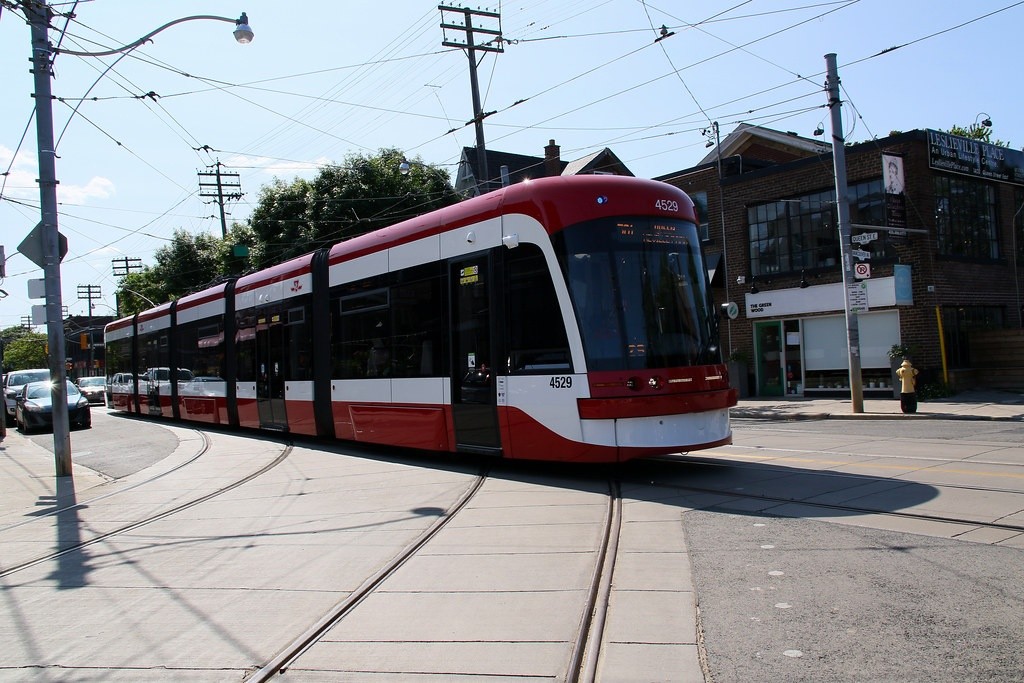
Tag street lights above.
[30,12,254,477]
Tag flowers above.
[886,343,911,360]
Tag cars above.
[109,367,195,395]
[183,377,226,397]
[7,380,91,433]
[3,369,50,416]
[75,377,105,404]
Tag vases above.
[890,358,904,399]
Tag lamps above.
[972,113,992,142]
[749,277,772,295]
[800,269,820,290]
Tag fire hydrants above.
[895,359,919,412]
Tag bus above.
[104,176,738,464]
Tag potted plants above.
[887,379,892,388]
[819,378,885,388]
